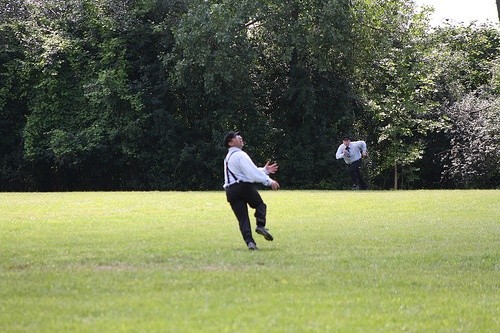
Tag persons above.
[336,136,368,190]
[223,130,281,248]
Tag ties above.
[345,146,350,157]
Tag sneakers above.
[255,226,273,241]
[247,241,257,250]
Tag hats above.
[224,130,240,145]
